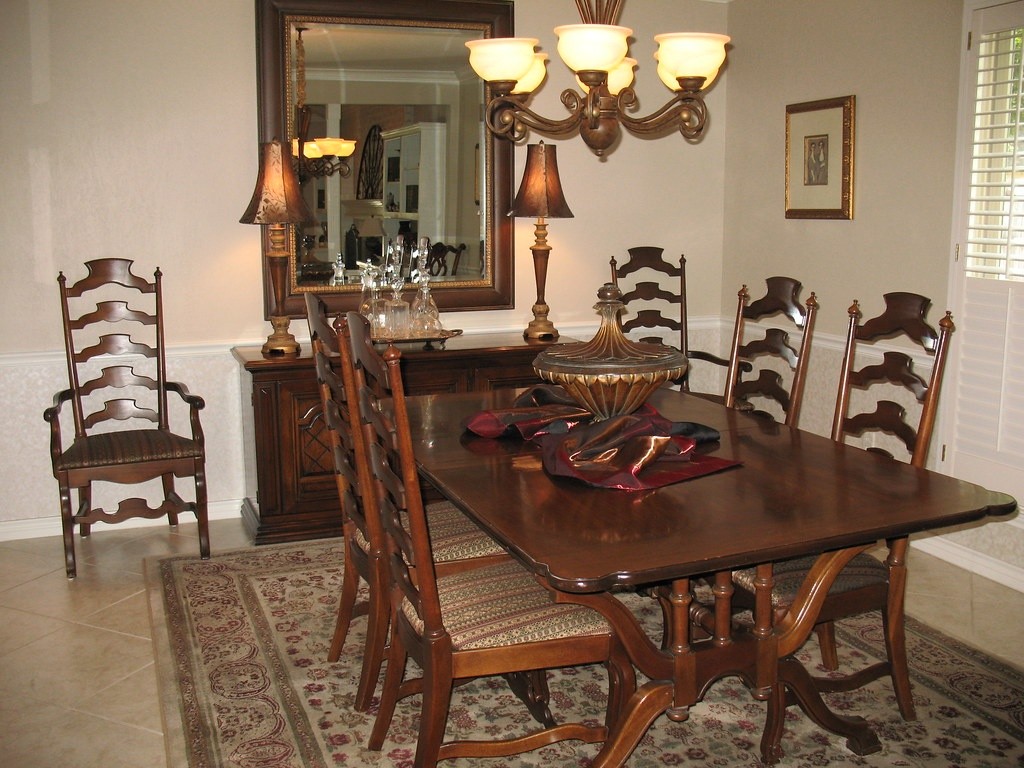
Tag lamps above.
[238,141,317,357]
[465,0,730,156]
[507,139,575,339]
[292,136,357,187]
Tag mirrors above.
[285,14,495,296]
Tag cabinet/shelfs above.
[379,122,447,267]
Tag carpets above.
[143,536,1023,768]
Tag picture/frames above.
[785,95,854,220]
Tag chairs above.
[385,242,466,283]
[689,277,820,640]
[730,292,953,766]
[44,257,210,578]
[305,287,549,713]
[343,311,638,768]
[610,245,755,415]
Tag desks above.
[376,387,1019,768]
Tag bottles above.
[357,273,442,339]
